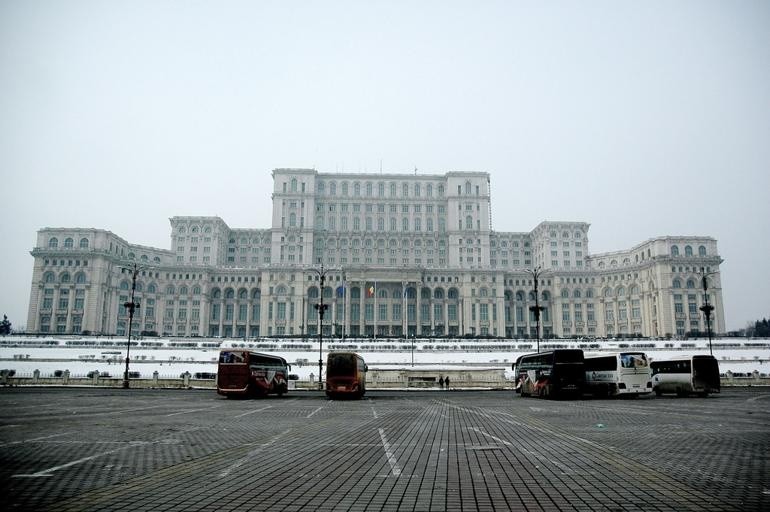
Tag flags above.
[336,286,344,295]
[368,286,375,297]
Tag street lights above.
[523,264,553,354]
[410,332,415,368]
[696,265,723,355]
[115,263,156,388]
[304,263,341,389]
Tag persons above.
[439,377,444,388]
[445,377,449,388]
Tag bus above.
[720,370,752,378]
[650,355,720,400]
[511,349,585,399]
[215,350,291,400]
[327,352,367,399]
[407,376,437,389]
[583,352,653,398]
